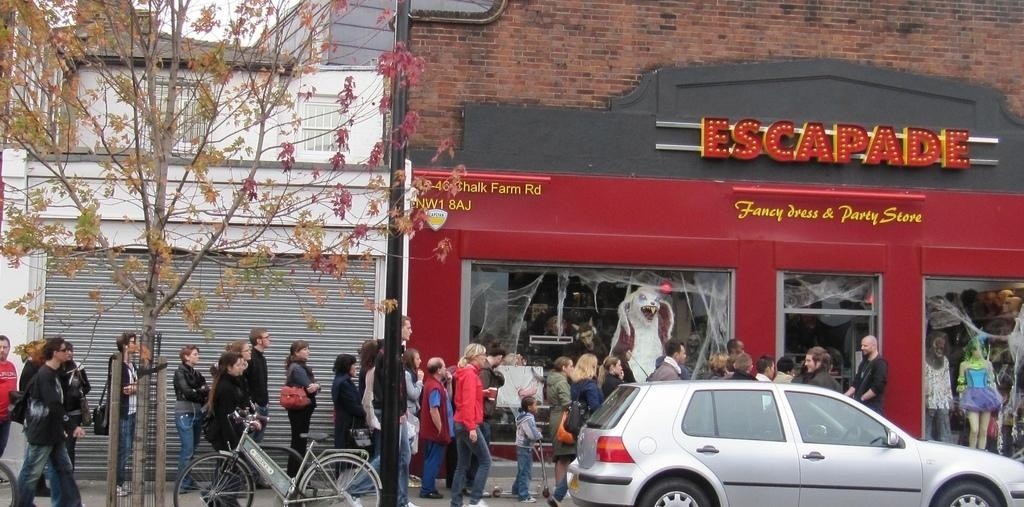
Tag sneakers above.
[116,483,131,497]
[419,491,443,499]
[342,491,363,507]
[401,502,420,507]
[450,489,491,507]
[520,496,535,505]
[547,497,561,507]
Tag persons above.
[647,338,841,393]
[546,342,635,505]
[287,340,321,487]
[844,335,888,414]
[956,337,1003,450]
[107,331,138,495]
[512,397,543,502]
[923,330,955,444]
[331,317,505,506]
[174,345,210,491]
[1,334,90,506]
[199,328,270,507]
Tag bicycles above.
[0,459,23,507]
[171,393,386,507]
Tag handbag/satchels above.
[349,413,371,448]
[280,384,311,409]
[556,400,591,445]
[200,401,211,439]
[7,389,30,425]
[987,413,999,439]
[92,403,111,436]
[80,396,92,427]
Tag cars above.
[475,356,554,444]
[563,377,1024,507]
